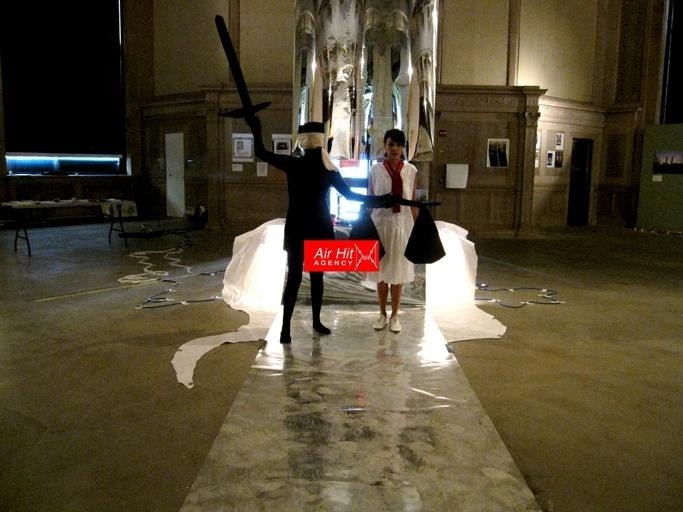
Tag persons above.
[244,114,398,344]
[282,331,331,507]
[365,129,419,333]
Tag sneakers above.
[389,315,402,332]
[373,314,389,330]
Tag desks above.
[1,196,138,258]
